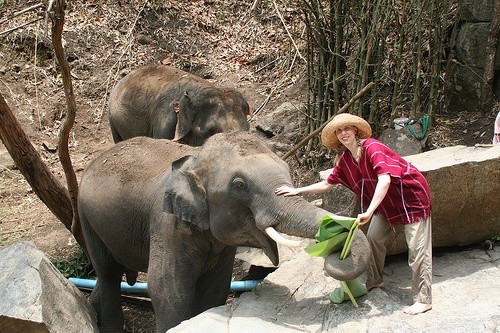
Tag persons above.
[274,113,434,316]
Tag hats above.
[320,113,372,150]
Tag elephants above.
[77,129,372,333]
[108,63,251,147]
